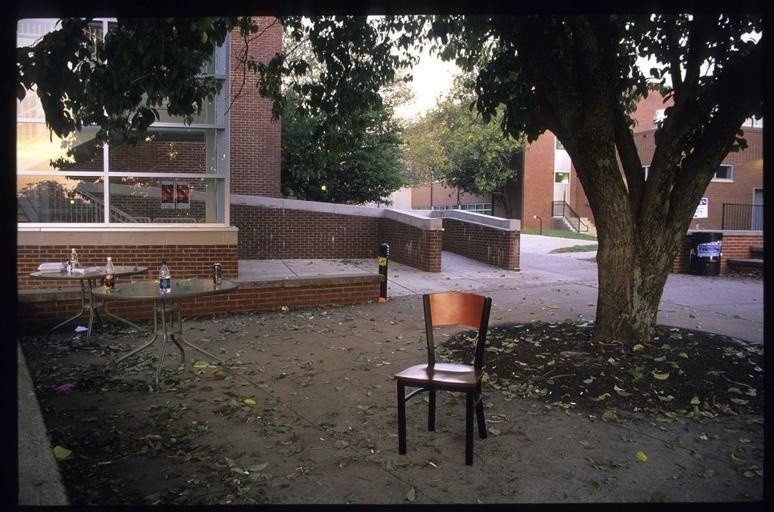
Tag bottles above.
[105,256,116,291]
[158,260,172,295]
[213,262,222,286]
[70,247,80,275]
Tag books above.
[37,262,66,273]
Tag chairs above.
[394,289,498,467]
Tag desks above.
[29,262,239,393]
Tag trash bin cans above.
[691,232,722,276]
